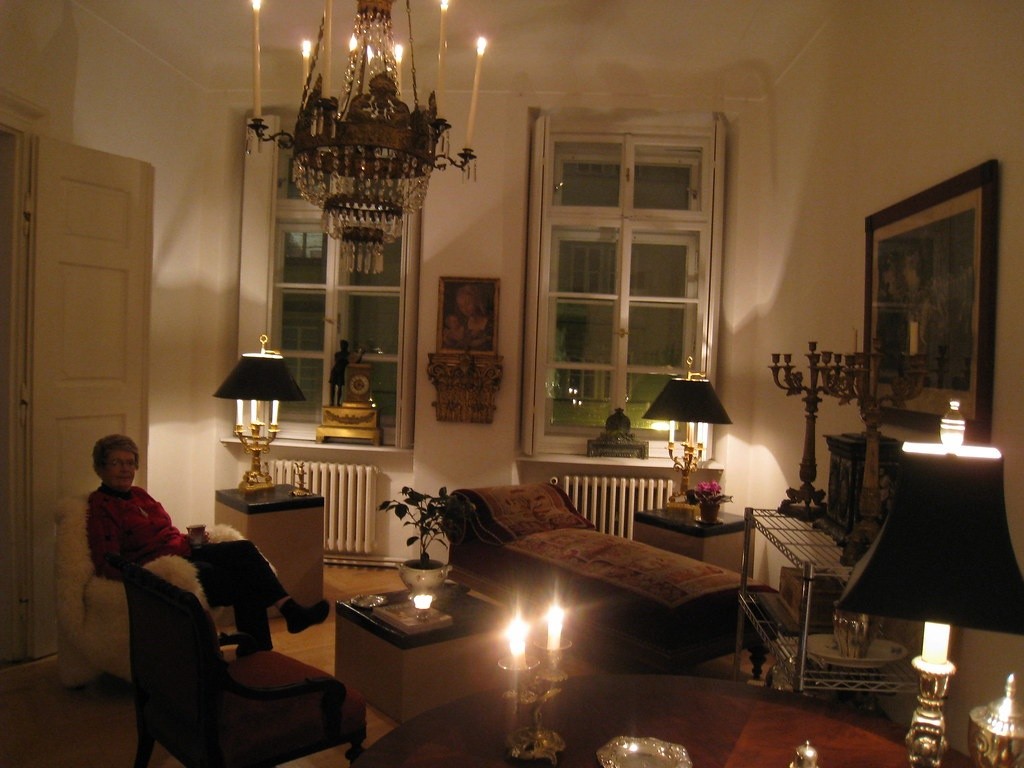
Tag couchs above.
[438,481,777,668]
[56,503,281,694]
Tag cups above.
[186,525,207,548]
[832,612,883,658]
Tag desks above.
[352,668,926,767]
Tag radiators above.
[258,458,380,557]
[547,476,676,542]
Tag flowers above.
[700,478,723,498]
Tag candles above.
[909,319,918,355]
[408,592,436,622]
[851,327,857,354]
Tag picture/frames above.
[861,157,1002,445]
[436,274,503,360]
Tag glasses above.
[106,458,136,468]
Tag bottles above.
[940,401,966,449]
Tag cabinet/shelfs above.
[730,504,943,702]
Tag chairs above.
[117,559,368,768]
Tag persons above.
[443,284,493,349]
[329,340,349,407]
[86,434,330,659]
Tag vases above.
[697,501,726,528]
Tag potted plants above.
[377,487,472,605]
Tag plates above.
[796,634,909,668]
[596,736,692,768]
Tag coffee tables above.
[334,589,497,726]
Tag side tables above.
[211,483,325,621]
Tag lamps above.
[242,2,487,275]
[494,584,574,767]
[212,331,307,495]
[835,398,1023,767]
[642,354,733,522]
